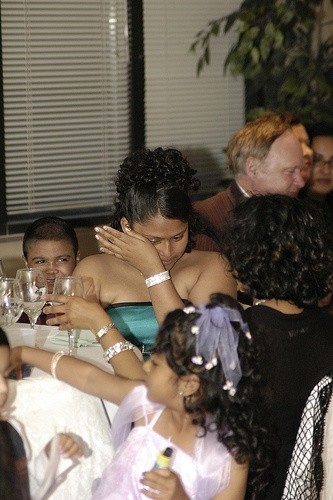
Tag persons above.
[188,113,333,303]
[5,293,281,500]
[43,193,333,500]
[281,353,333,500]
[16,216,81,325]
[60,146,238,362]
[0,326,80,500]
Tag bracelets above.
[145,269,172,292]
[102,340,134,364]
[49,352,63,379]
[94,321,116,341]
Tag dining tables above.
[0,323,145,500]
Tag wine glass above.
[14,268,48,348]
[0,278,24,329]
[52,276,83,357]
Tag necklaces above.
[254,297,267,305]
